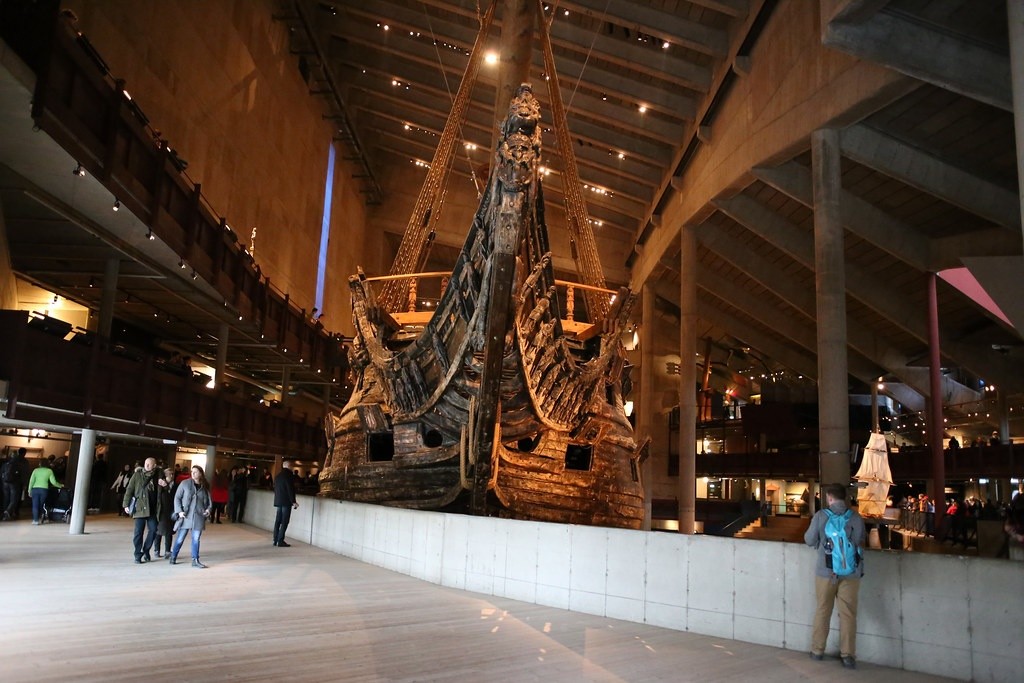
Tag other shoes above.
[170,558,176,565]
[842,656,856,669]
[2,511,11,521]
[143,547,151,562]
[273,541,291,547]
[164,551,172,559]
[32,520,39,525]
[216,520,222,524]
[210,517,215,523]
[809,651,823,660]
[155,551,160,558]
[134,558,142,564]
[191,559,205,568]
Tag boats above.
[318,82,646,533]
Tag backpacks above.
[821,507,864,575]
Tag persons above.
[262,466,321,491]
[804,481,866,669]
[886,431,1024,516]
[0,446,252,565]
[273,461,296,548]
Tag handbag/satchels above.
[171,512,179,521]
